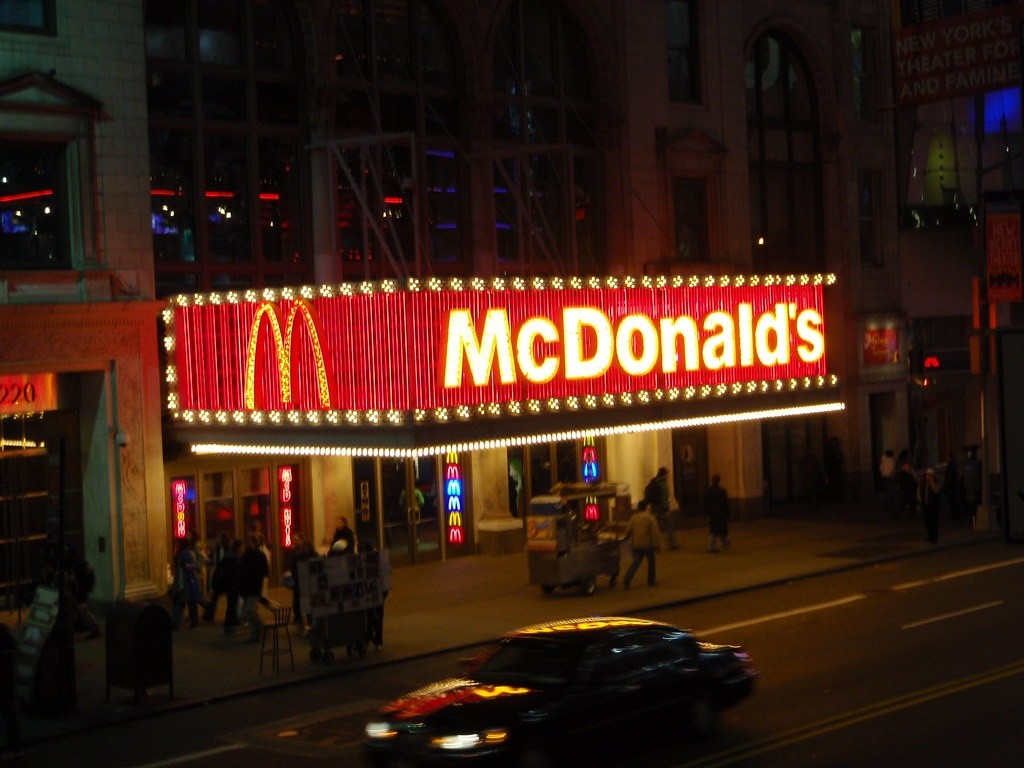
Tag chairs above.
[259,608,295,676]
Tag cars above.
[364,615,758,768]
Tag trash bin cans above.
[105,601,174,704]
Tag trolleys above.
[295,552,392,660]
[523,482,634,594]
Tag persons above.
[509,467,518,518]
[623,498,661,591]
[645,467,681,551]
[44,544,102,641]
[924,462,941,543]
[399,478,424,545]
[704,474,732,554]
[0,623,23,759]
[166,516,385,652]
[798,436,1004,517]
